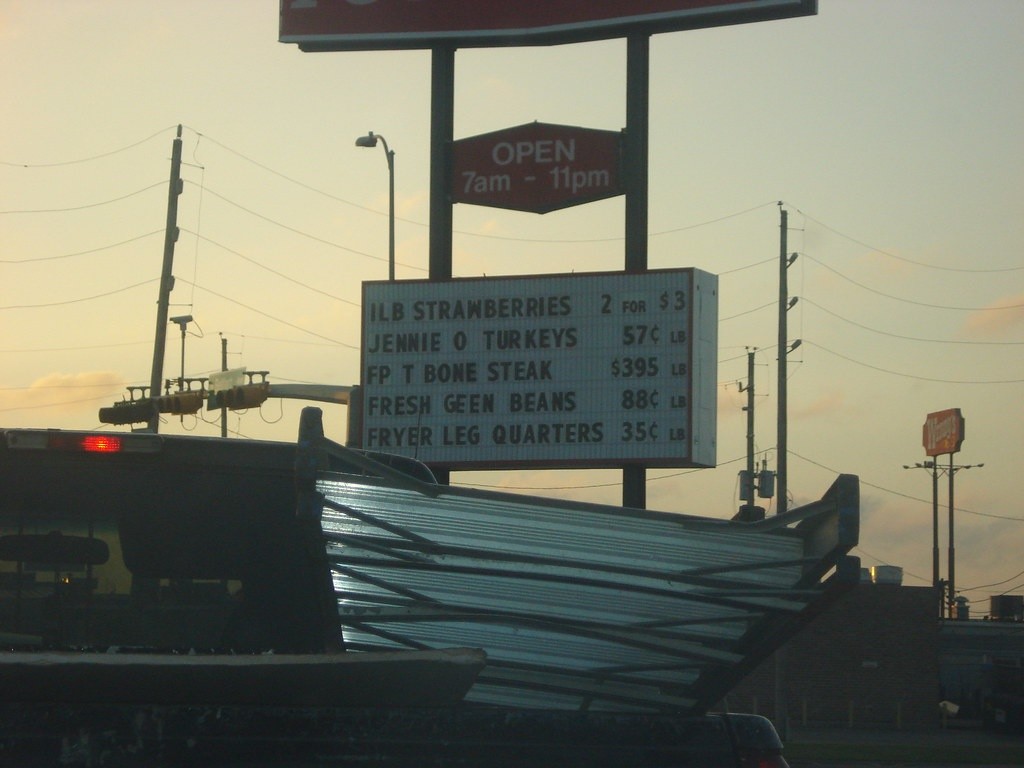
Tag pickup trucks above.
[1,404,795,767]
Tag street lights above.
[354,130,397,279]
